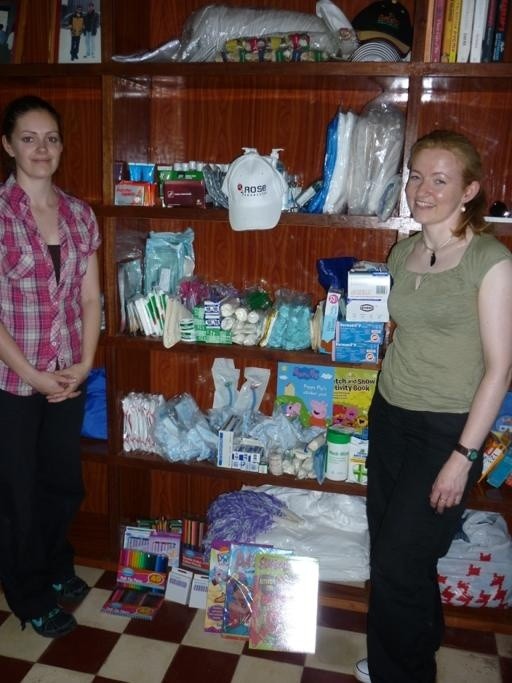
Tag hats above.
[351,0,413,59]
[221,153,289,231]
[316,1,360,61]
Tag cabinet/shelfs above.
[0,0,111,575]
[404,0,512,634]
[100,0,420,609]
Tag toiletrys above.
[127,160,229,209]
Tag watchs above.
[454,442,479,462]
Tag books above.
[423,0,508,63]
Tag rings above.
[438,498,447,504]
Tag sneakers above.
[25,601,77,638]
[353,658,372,683]
[49,576,90,608]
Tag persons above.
[1,97,103,637]
[351,128,511,683]
[67,4,87,61]
[83,3,100,58]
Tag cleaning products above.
[266,145,292,211]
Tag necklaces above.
[422,235,452,266]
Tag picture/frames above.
[54,0,101,64]
[0,0,28,64]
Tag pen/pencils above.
[122,537,176,573]
[182,555,209,570]
[194,585,208,592]
[152,514,168,534]
[104,575,164,608]
